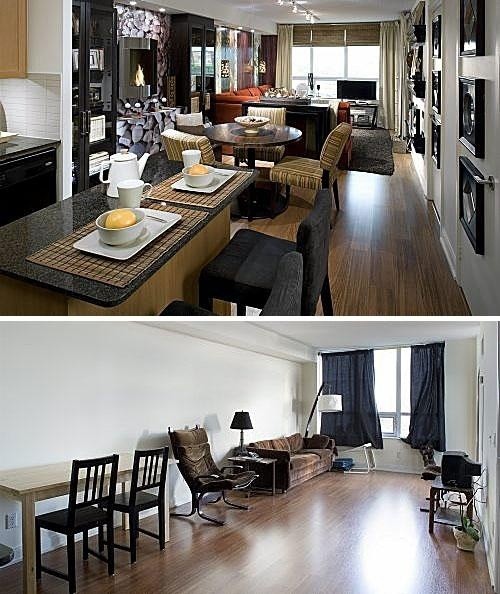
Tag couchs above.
[232,433,337,492]
[215,84,353,168]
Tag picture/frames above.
[431,0,486,255]
[73,48,100,69]
[89,115,105,142]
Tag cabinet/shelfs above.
[171,13,216,122]
[0,148,58,227]
[71,0,117,192]
[1,0,27,77]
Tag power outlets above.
[7,512,17,528]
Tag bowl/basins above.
[181,166,218,189]
[94,208,146,248]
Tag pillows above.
[307,434,329,449]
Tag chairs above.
[160,127,215,162]
[269,121,352,228]
[34,452,120,594]
[168,424,260,524]
[176,111,223,150]
[97,444,168,564]
[159,251,305,314]
[233,105,286,191]
[199,187,333,315]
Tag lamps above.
[277,0,315,25]
[259,34,266,72]
[221,28,230,76]
[305,382,342,436]
[229,410,253,453]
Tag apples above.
[106,209,135,229]
[187,164,208,175]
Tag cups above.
[181,149,201,166]
[117,179,154,211]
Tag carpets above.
[337,126,394,174]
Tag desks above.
[0,453,179,591]
[428,476,473,532]
[206,122,303,219]
[227,456,277,498]
[350,103,378,129]
[0,158,260,315]
[241,99,332,164]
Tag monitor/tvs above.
[337,80,376,100]
[441,451,481,487]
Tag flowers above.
[440,488,481,542]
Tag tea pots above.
[97,149,151,199]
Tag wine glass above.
[317,85,321,97]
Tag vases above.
[452,524,481,552]
